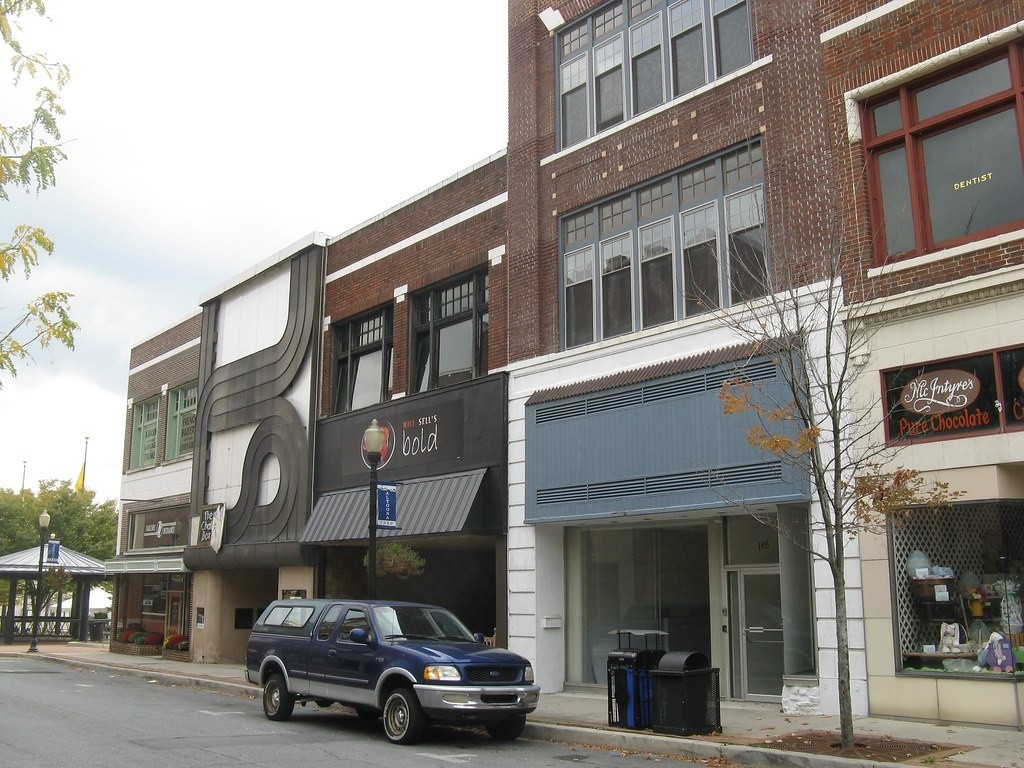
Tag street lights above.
[364,419,387,599]
[28,509,51,651]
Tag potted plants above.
[365,539,427,579]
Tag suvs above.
[244,597,542,746]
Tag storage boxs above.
[911,578,956,601]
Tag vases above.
[179,646,190,651]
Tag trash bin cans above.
[89,622,104,642]
[606,647,666,729]
[658,651,709,735]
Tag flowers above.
[962,588,988,601]
[179,640,189,646]
[992,578,1021,596]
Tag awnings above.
[299,468,493,546]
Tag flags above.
[75,464,84,492]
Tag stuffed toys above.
[977,631,1016,672]
[942,630,961,653]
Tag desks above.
[902,651,978,658]
